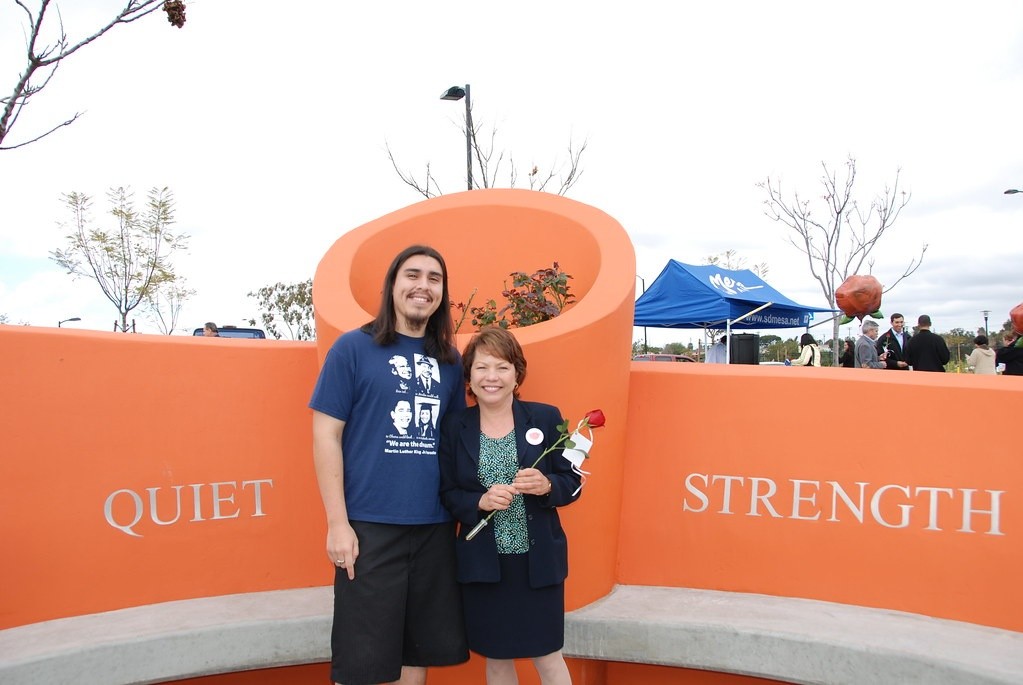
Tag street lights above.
[440,82,476,191]
[58,317,81,328]
[980,310,991,341]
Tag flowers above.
[883,331,895,357]
[466,409,606,540]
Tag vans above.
[192,326,266,340]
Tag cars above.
[633,353,696,362]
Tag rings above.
[336,560,345,565]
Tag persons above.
[791,333,821,367]
[203,322,220,337]
[965,335,996,374]
[840,341,855,367]
[995,331,1023,377]
[905,315,949,372]
[309,246,467,685]
[704,335,727,364]
[877,313,912,370]
[855,320,887,369]
[439,329,581,685]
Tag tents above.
[634,258,843,364]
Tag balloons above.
[1010,302,1023,348]
[836,275,884,324]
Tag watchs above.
[548,483,552,491]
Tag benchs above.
[0,585,334,685]
[562,585,1023,685]
